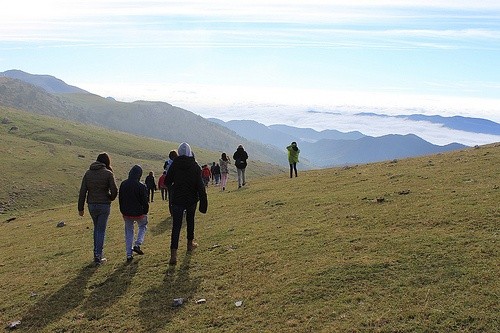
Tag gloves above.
[199,196,207,214]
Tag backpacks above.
[173,182,193,208]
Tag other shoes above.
[132,245,144,255]
[170,255,177,264]
[187,244,197,253]
[94,256,101,264]
[101,258,107,263]
[127,254,133,261]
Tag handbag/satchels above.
[235,161,247,169]
[226,167,231,174]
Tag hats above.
[177,142,191,156]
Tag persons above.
[77,152,118,263]
[163,142,208,251]
[145,144,248,203]
[118,165,149,263]
[286,142,300,178]
